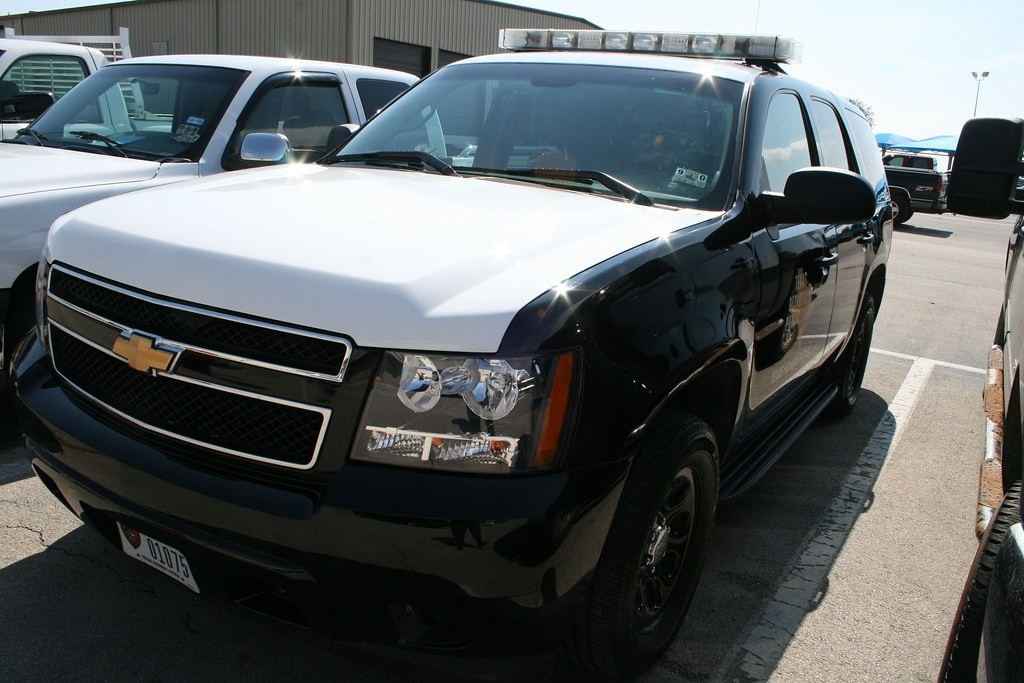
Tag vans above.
[881,153,938,170]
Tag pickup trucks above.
[0,38,176,144]
[883,165,951,226]
[0,53,561,372]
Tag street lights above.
[972,71,990,118]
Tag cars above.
[9,27,900,682]
[931,117,1024,683]
